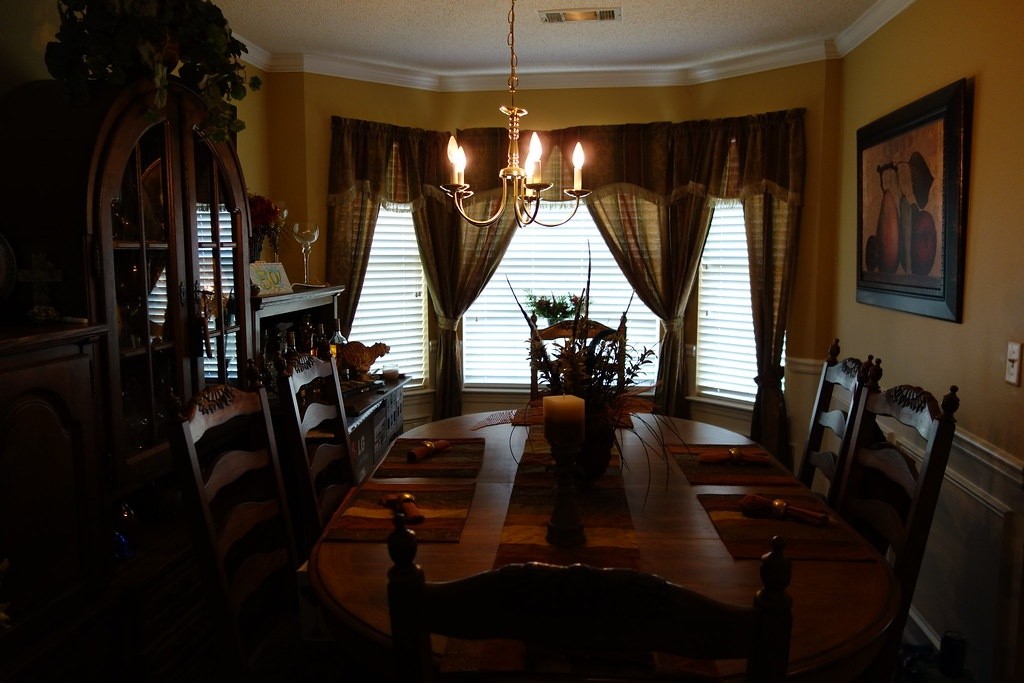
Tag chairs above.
[836,359,960,683]
[386,508,792,683]
[271,334,360,539]
[163,359,371,683]
[798,336,873,507]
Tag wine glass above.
[294,223,320,284]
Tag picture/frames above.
[856,78,967,322]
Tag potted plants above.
[45,0,262,143]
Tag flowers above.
[247,195,283,234]
[519,299,693,508]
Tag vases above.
[250,237,262,262]
[574,429,616,488]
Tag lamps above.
[440,1,593,228]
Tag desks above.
[309,405,900,683]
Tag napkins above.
[700,448,770,464]
[744,494,836,527]
[406,439,452,462]
[379,491,425,523]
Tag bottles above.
[301,314,351,380]
[261,328,308,406]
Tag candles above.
[542,393,585,445]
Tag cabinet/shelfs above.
[0,80,405,683]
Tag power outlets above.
[1006,342,1023,387]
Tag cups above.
[383,364,398,386]
